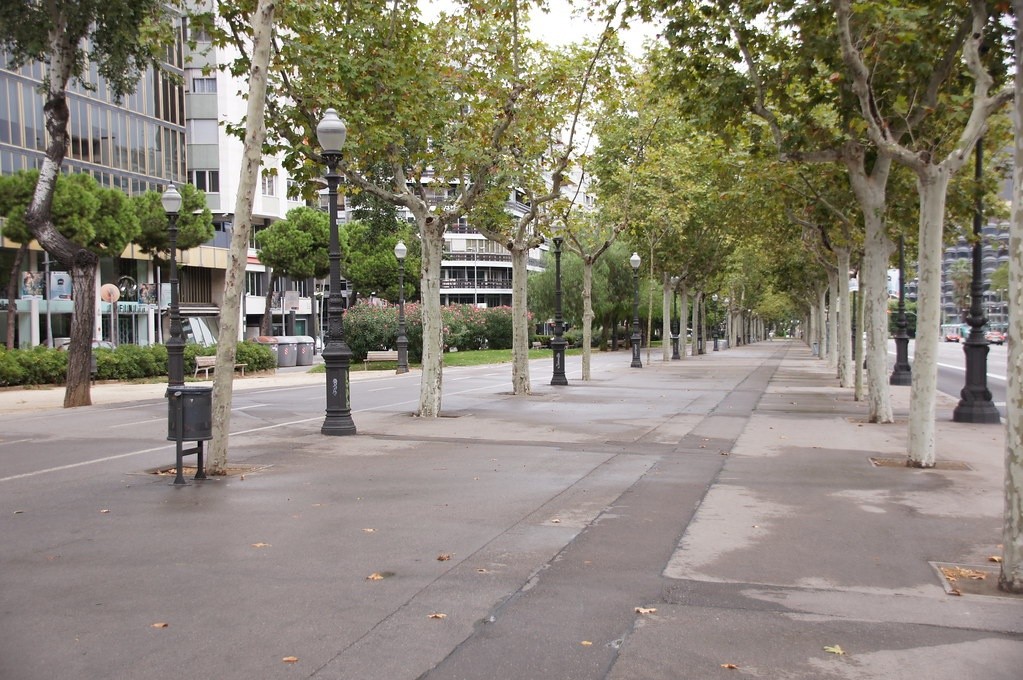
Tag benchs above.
[363,350,408,371]
[193,356,248,379]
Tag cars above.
[986,332,1004,345]
[945,332,960,343]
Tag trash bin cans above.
[253,336,279,367]
[166,385,213,441]
[272,336,298,367]
[296,336,315,366]
[812,342,818,355]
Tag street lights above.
[630,252,643,368]
[395,240,410,375]
[160,179,186,398]
[671,273,680,360]
[317,108,357,435]
[550,220,568,385]
[712,294,719,350]
